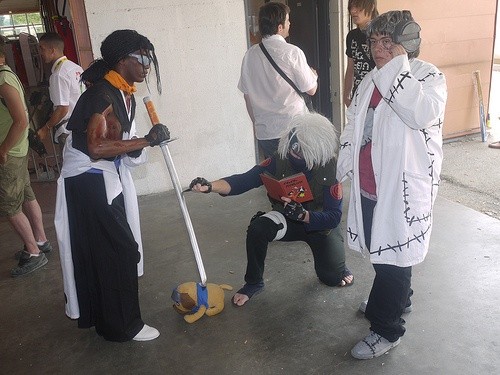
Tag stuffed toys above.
[171,281,233,323]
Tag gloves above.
[145,124,170,146]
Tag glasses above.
[128,53,152,66]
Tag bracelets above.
[46,123,52,129]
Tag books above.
[259,171,313,204]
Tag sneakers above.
[359,299,412,313]
[8,251,48,277]
[351,332,400,359]
[15,239,52,259]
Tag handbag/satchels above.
[301,92,313,112]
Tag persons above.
[190,113,354,307]
[35,32,87,153]
[237,2,318,161]
[343,0,378,107]
[53,29,170,342]
[0,35,53,276]
[336,10,447,360]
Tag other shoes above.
[133,324,160,341]
